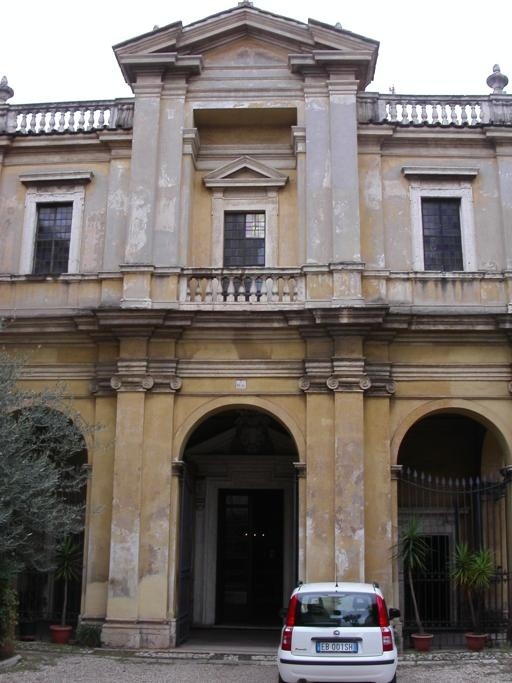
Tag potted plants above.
[47,531,82,643]
[388,511,498,652]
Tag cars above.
[277,578,402,683]
